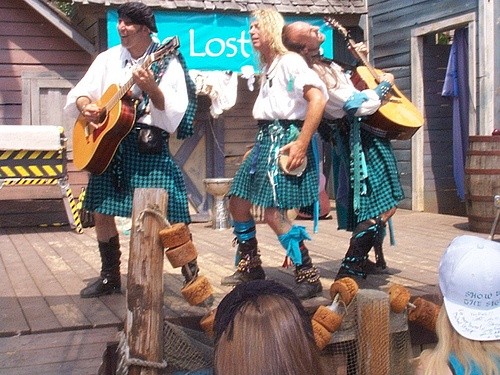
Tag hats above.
[118,2,158,33]
[438,235,500,341]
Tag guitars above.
[323,14,424,141]
[298,173,331,219]
[72,35,181,176]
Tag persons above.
[221,9,329,301]
[64,1,214,308]
[282,21,404,282]
[411,236,500,375]
[212,279,321,375]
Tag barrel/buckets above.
[465,135,500,234]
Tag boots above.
[293,241,323,299]
[221,237,266,286]
[181,233,213,306]
[80,234,121,298]
[334,223,387,289]
